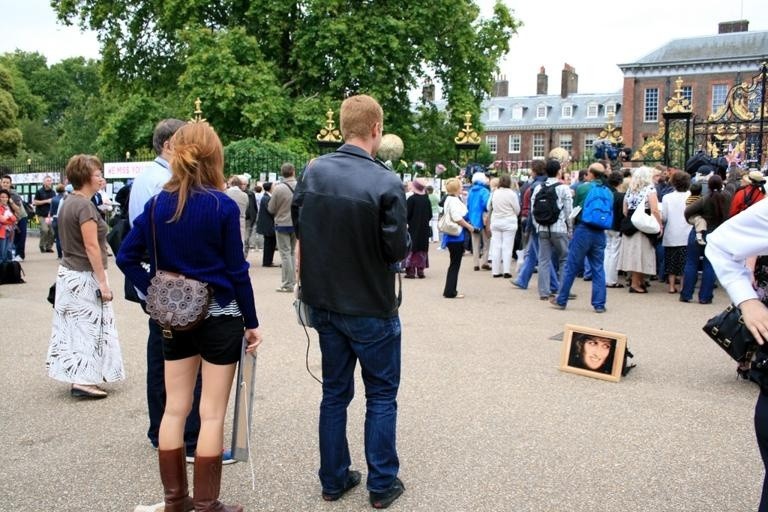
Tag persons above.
[393,166,766,314]
[114,116,263,512]
[565,331,618,377]
[124,116,241,467]
[44,152,128,402]
[1,175,28,261]
[704,192,767,512]
[34,175,113,256]
[288,91,413,510]
[116,163,297,292]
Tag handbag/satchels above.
[430,217,439,243]
[293,280,316,327]
[147,269,209,339]
[46,282,58,308]
[703,300,763,363]
[45,212,53,224]
[438,214,462,237]
[482,211,492,240]
[631,190,664,235]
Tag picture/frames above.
[559,321,625,384]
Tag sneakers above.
[583,273,719,305]
[320,470,362,501]
[473,259,512,278]
[56,257,62,261]
[184,441,239,465]
[262,261,279,267]
[396,268,426,278]
[540,291,577,310]
[275,286,294,292]
[40,246,54,253]
[510,279,528,289]
[368,477,404,509]
[594,306,606,313]
[436,244,488,258]
[11,248,24,262]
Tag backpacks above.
[580,182,614,230]
[22,200,35,219]
[568,205,582,219]
[532,183,561,226]
[1,261,25,285]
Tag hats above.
[742,170,767,188]
[408,179,427,195]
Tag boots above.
[156,444,194,512]
[191,447,244,511]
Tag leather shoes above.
[70,382,108,399]
[444,291,464,298]
